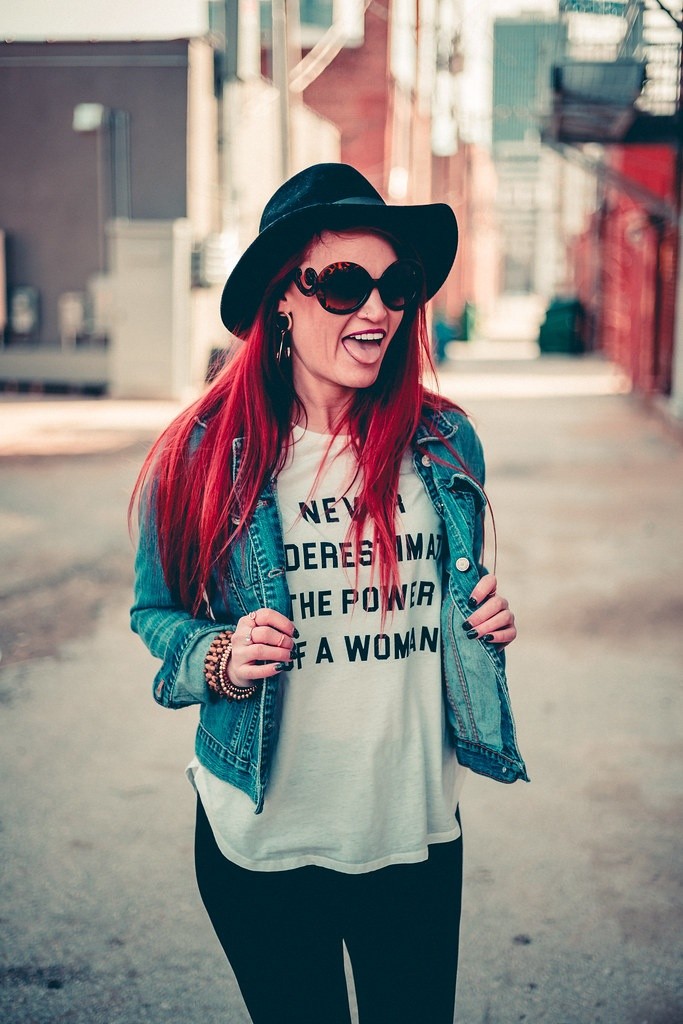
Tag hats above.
[221,163,458,342]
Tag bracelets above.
[204,630,259,704]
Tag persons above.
[128,163,530,1024]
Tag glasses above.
[291,259,426,314]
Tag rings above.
[246,627,254,643]
[489,590,496,595]
[249,611,256,626]
[278,632,284,646]
